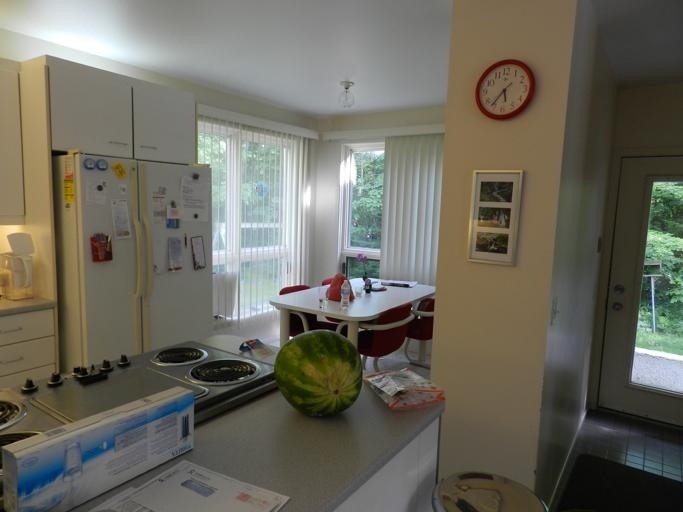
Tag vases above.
[363,274,368,280]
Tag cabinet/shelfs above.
[1,303,61,389]
[20,53,198,165]
[0,61,25,225]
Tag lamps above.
[335,81,356,107]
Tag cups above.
[62,442,84,482]
[318,297,329,311]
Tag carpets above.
[553,450,683,510]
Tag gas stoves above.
[154,344,259,387]
[0,402,67,470]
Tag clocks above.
[474,58,535,120]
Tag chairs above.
[265,274,436,374]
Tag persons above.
[487,238,499,251]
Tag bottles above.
[339,279,352,309]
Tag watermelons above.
[274,329,362,418]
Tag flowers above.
[355,253,368,274]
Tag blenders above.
[0,232,35,301]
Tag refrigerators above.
[51,152,214,373]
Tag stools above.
[431,464,555,511]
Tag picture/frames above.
[465,168,524,268]
